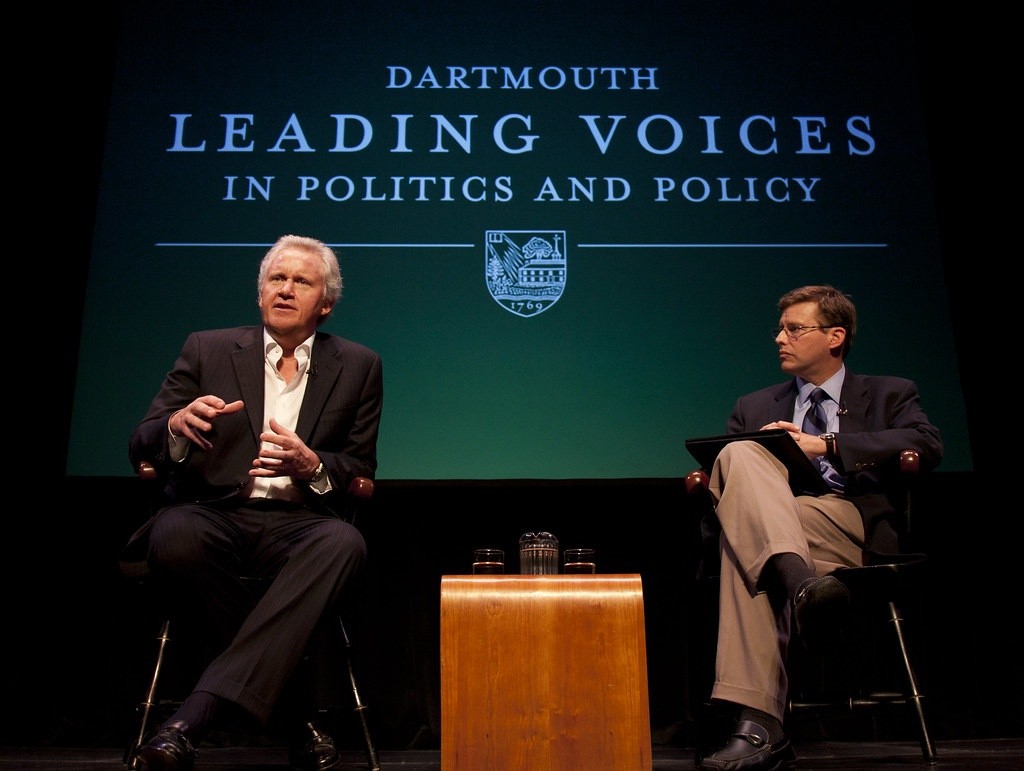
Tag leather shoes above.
[297,721,341,771]
[702,721,799,771]
[129,720,199,771]
[793,576,850,650]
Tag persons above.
[698,284,946,771]
[126,231,385,771]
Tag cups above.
[471,549,505,574]
[563,549,596,574]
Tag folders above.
[685,428,832,493]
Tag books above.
[684,426,833,498]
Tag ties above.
[802,387,846,494]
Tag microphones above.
[306,368,317,375]
[837,409,845,415]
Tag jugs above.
[519,532,559,574]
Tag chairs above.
[685,448,938,766]
[127,461,379,770]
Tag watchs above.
[817,431,836,457]
[306,459,326,484]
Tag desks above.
[441,574,652,771]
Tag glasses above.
[772,326,832,339]
